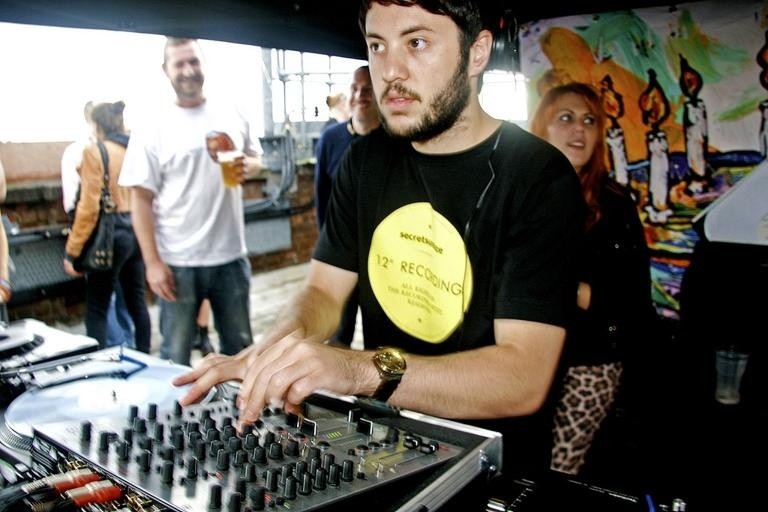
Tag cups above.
[216,149,245,189]
[713,348,752,406]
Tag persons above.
[529,81,650,479]
[118,36,265,370]
[154,288,218,359]
[314,65,384,349]
[63,101,152,354]
[171,0,586,473]
[0,160,14,321]
[60,99,137,348]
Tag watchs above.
[371,346,407,405]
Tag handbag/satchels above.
[65,140,114,274]
